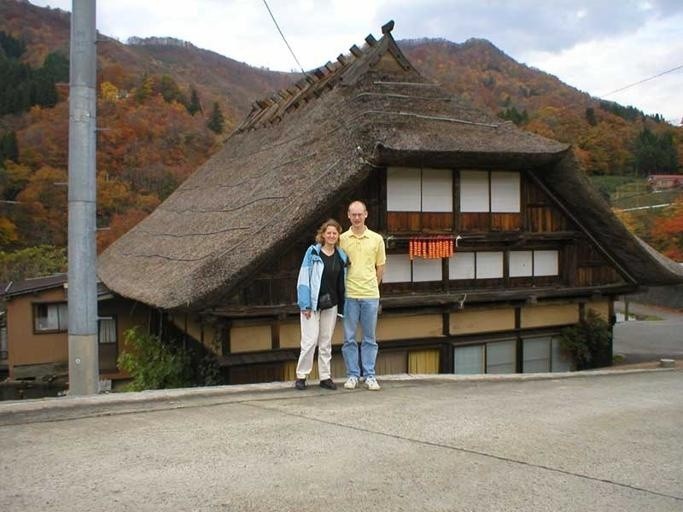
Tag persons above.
[295,219,348,390]
[337,201,387,391]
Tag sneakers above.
[344,376,358,389]
[320,379,337,390]
[365,375,381,390]
[296,379,306,388]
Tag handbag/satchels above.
[319,292,332,309]
